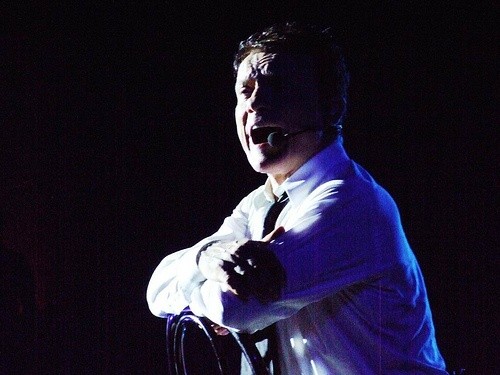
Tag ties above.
[262,199,290,239]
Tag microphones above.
[267,125,329,147]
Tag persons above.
[146,21,449,375]
